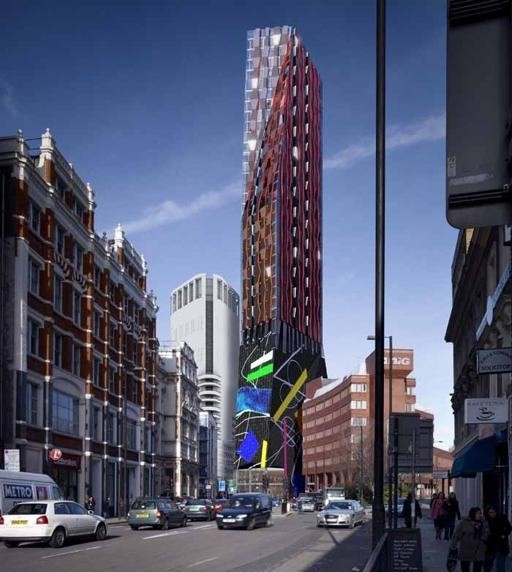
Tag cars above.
[385,495,409,517]
[127,496,229,531]
[269,496,317,513]
[1,500,108,549]
[316,499,366,529]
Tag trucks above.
[313,486,346,511]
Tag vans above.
[0,469,68,525]
[216,492,273,531]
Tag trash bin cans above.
[282,498,287,515]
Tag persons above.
[442,492,462,544]
[431,492,447,542]
[474,502,511,572]
[449,506,490,572]
[88,495,96,513]
[430,493,438,507]
[400,490,422,528]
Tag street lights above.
[365,334,395,526]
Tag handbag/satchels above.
[447,546,458,572]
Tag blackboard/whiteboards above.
[385,528,423,572]
